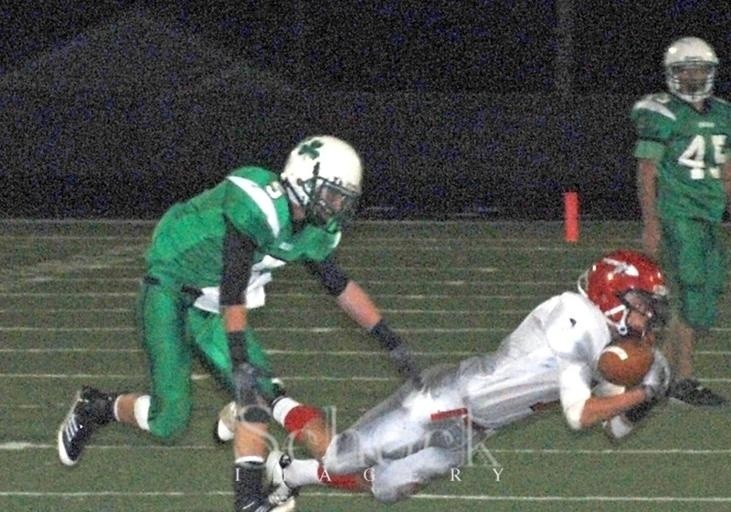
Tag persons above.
[55,133,424,512]
[263,248,672,512]
[627,36,730,410]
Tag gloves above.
[387,342,423,392]
[231,359,277,409]
[641,347,672,404]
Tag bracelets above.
[228,330,251,367]
[364,317,404,351]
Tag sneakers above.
[665,378,726,411]
[57,384,102,467]
[213,376,287,445]
[263,448,292,495]
[233,483,297,512]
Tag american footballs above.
[599,336,654,386]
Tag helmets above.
[576,250,670,342]
[664,35,719,103]
[279,134,363,234]
